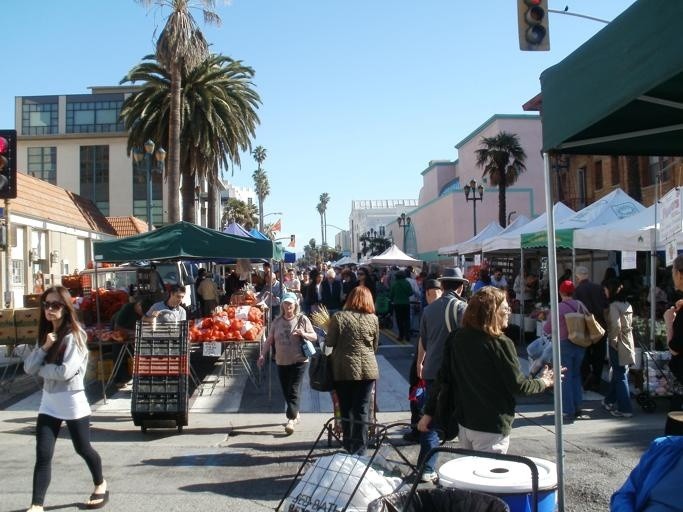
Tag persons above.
[24,287,109,512]
[109,250,683,512]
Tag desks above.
[84,339,135,403]
[204,323,266,396]
[508,313,526,327]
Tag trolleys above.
[274,417,426,512]
[633,328,683,413]
[141,309,182,433]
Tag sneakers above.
[600,399,614,411]
[295,413,301,423]
[609,411,632,417]
[419,470,438,482]
[285,421,295,434]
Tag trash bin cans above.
[366,487,512,512]
[433,454,558,512]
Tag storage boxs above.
[1,308,41,344]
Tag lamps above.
[51,250,58,263]
[29,248,37,262]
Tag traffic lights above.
[517,0,550,51]
[0,129,18,200]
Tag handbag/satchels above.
[577,301,605,343]
[564,303,592,347]
[408,378,426,402]
[297,315,316,357]
[309,339,333,392]
[430,333,459,441]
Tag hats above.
[282,291,298,304]
[559,280,576,294]
[437,266,469,285]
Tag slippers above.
[87,490,109,509]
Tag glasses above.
[43,301,62,311]
[500,306,512,315]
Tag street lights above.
[464,177,483,237]
[133,137,167,232]
[367,228,377,256]
[397,213,411,254]
